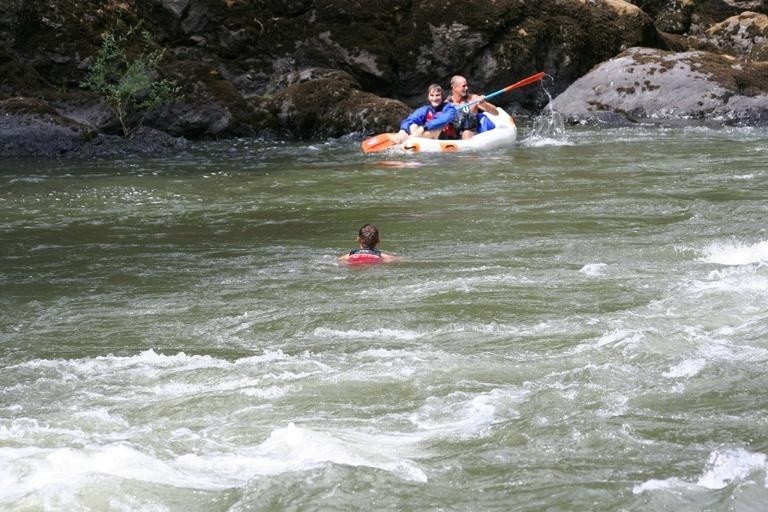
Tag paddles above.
[363,71,545,153]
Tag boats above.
[398,102,517,156]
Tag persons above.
[443,75,499,140]
[339,224,400,266]
[396,84,462,140]
